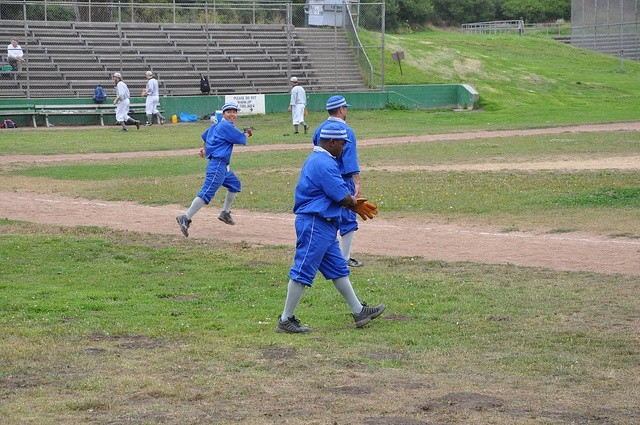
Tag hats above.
[326,94,352,110]
[220,102,239,112]
[290,76,298,82]
[146,70,153,79]
[113,71,122,78]
[320,122,352,142]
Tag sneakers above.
[121,128,128,132]
[144,121,152,125]
[345,256,364,268]
[274,315,312,334]
[135,119,140,128]
[353,302,386,328]
[218,209,236,225]
[159,115,165,124]
[175,213,192,237]
[304,124,309,134]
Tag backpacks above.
[200,76,210,91]
[92,84,107,101]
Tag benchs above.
[0,104,37,128]
[34,102,166,128]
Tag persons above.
[311,96,365,270]
[287,77,310,134]
[143,71,166,127]
[8,39,25,79]
[276,122,386,334]
[113,72,140,132]
[516,15,525,34]
[176,103,253,237]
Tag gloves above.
[352,196,378,222]
[244,128,252,135]
